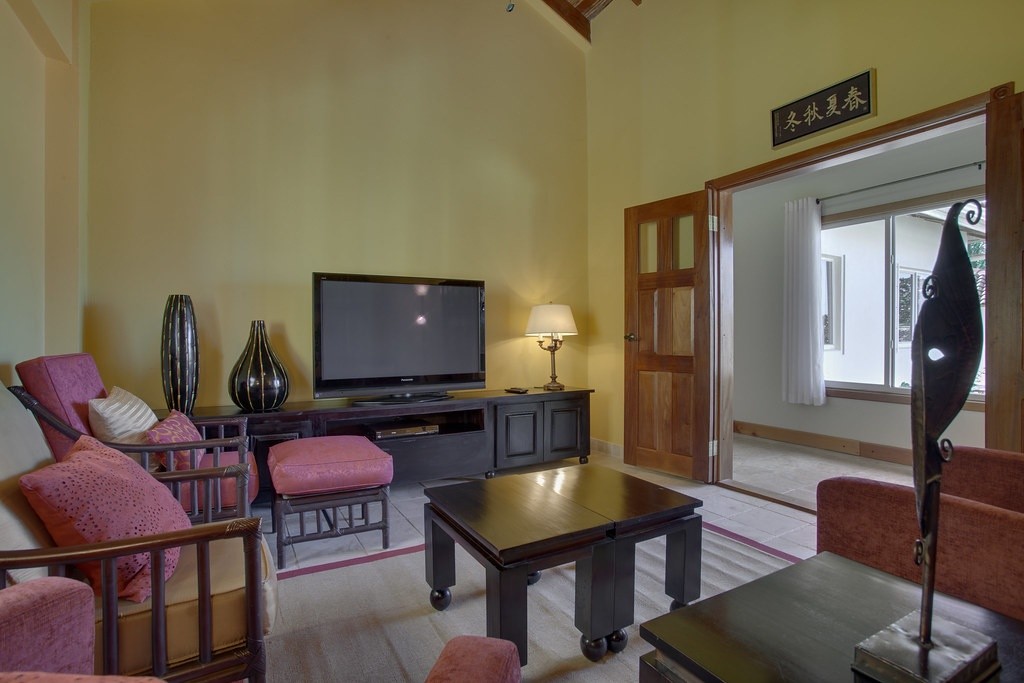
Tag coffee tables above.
[524,462,703,655]
[422,474,615,667]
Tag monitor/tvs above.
[311,271,486,404]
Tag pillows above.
[16,433,191,604]
[86,386,161,467]
[144,407,206,472]
[268,435,394,498]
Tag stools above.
[270,481,391,569]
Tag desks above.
[637,550,1023,683]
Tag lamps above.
[525,300,579,391]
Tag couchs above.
[0,574,527,683]
[816,445,1022,621]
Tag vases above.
[160,294,199,417]
[226,319,289,413]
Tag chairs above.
[0,383,268,683]
[8,384,248,523]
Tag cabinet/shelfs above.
[153,385,596,506]
